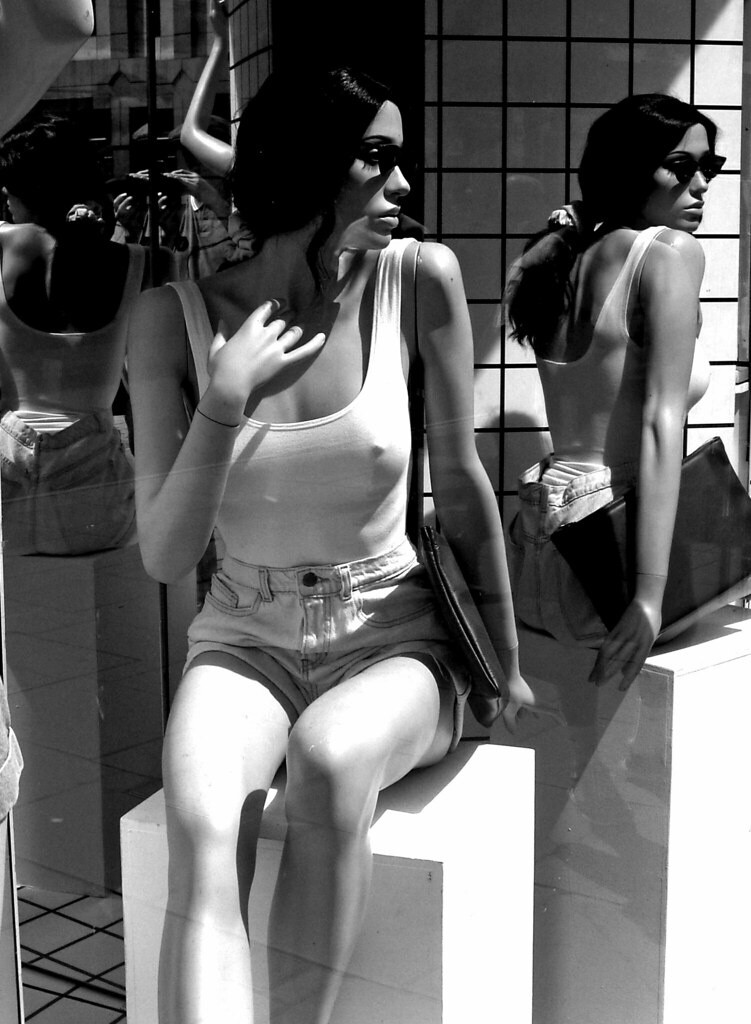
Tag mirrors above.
[0,0,751,1024]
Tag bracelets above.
[196,409,239,428]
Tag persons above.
[126,66,536,1024]
[499,92,730,696]
[0,114,177,559]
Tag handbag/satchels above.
[550,437,751,646]
[420,526,510,729]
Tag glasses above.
[356,142,416,179]
[664,152,726,185]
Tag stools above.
[3,532,191,896]
[119,742,535,1024]
[488,605,751,1024]
